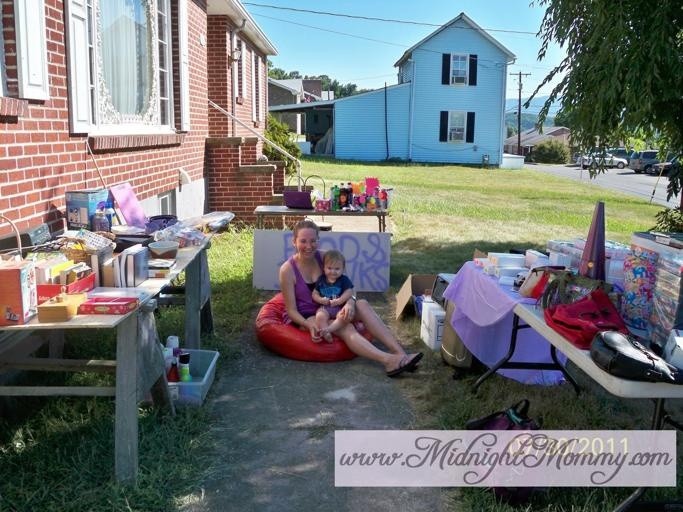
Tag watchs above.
[349,294,356,302]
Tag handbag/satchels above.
[145,215,177,235]
[465,399,544,504]
[520,265,678,384]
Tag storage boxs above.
[420,300,448,351]
[395,273,437,320]
[65,189,113,231]
[0,260,38,326]
[168,349,219,407]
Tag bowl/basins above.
[148,241,179,260]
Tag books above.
[101,242,177,287]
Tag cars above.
[570,139,681,178]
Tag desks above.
[465,262,683,511]
[253,206,388,233]
[0,227,222,486]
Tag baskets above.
[60,231,118,268]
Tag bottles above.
[92,201,116,232]
[330,182,394,210]
[166,337,193,382]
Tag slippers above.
[387,352,424,377]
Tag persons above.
[311,249,354,343]
[279,220,424,377]
